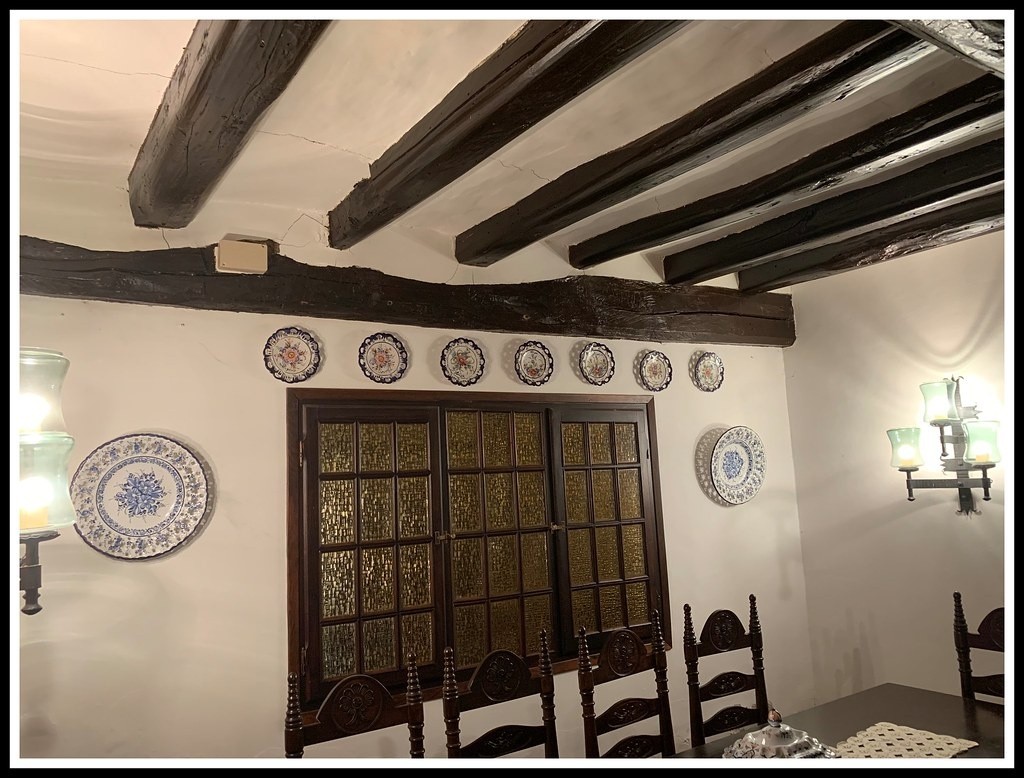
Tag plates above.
[582,341,614,385]
[358,331,409,383]
[516,339,554,385]
[264,327,321,385]
[438,336,486,386]
[640,352,675,393]
[709,427,765,506]
[70,434,209,562]
[695,351,726,392]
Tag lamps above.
[886,376,996,515]
[19,344,75,613]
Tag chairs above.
[683,593,770,748]
[577,609,677,758]
[285,651,426,759]
[951,590,1005,703]
[443,627,561,759]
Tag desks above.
[664,683,1004,759]
